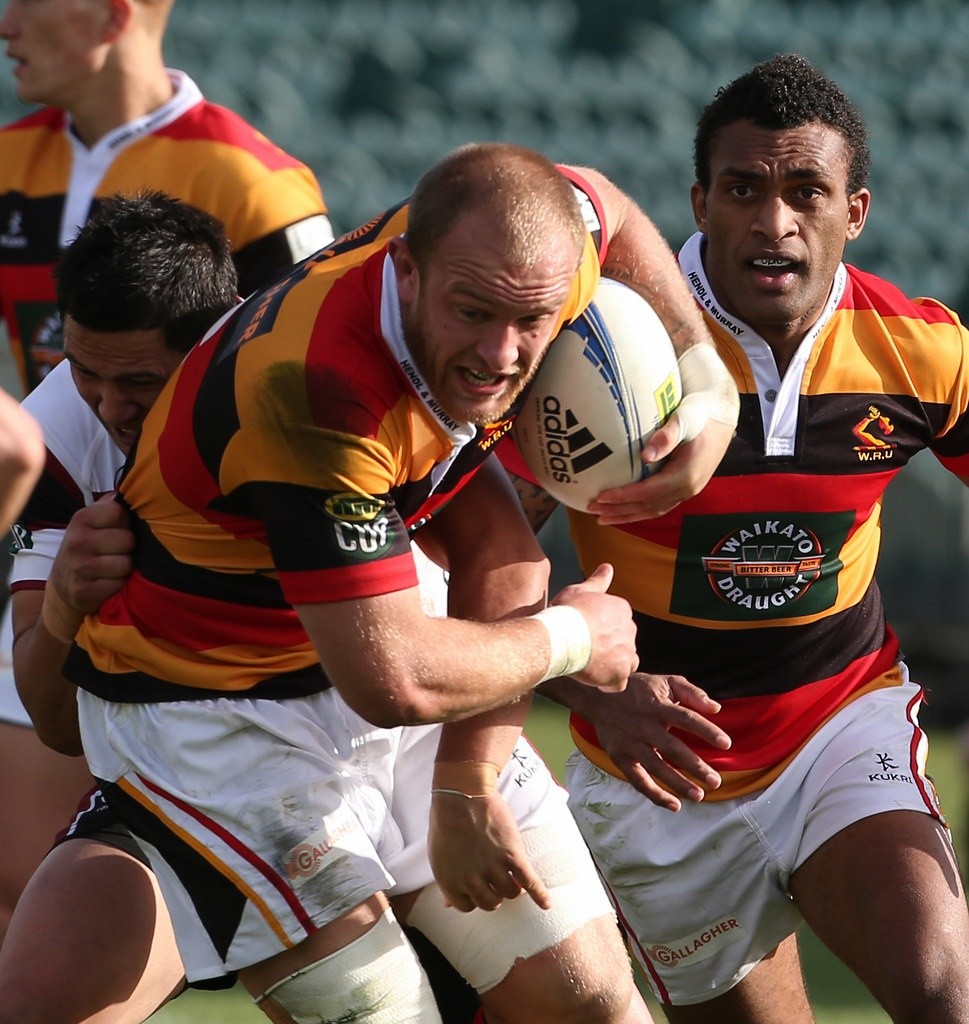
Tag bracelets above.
[430,760,501,798]
[42,579,82,644]
[530,605,590,686]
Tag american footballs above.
[512,275,688,514]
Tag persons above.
[508,55,969,1024]
[0,387,46,539]
[0,0,334,394]
[0,192,653,1024]
[63,142,741,1024]
